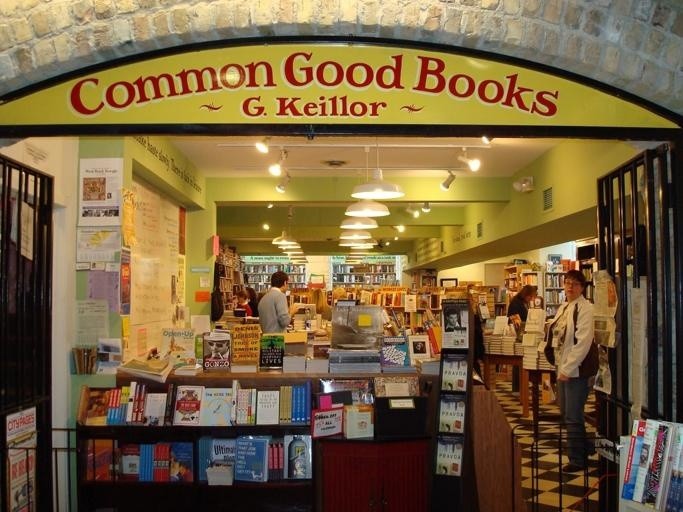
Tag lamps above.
[253,132,494,265]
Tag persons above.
[545,270,597,471]
[506,286,537,393]
[442,307,461,330]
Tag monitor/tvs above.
[337,300,360,305]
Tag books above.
[214,248,443,325]
[504,252,596,320]
[481,309,557,370]
[618,418,682,511]
[71,326,312,480]
[311,326,439,438]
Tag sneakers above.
[562,463,587,472]
